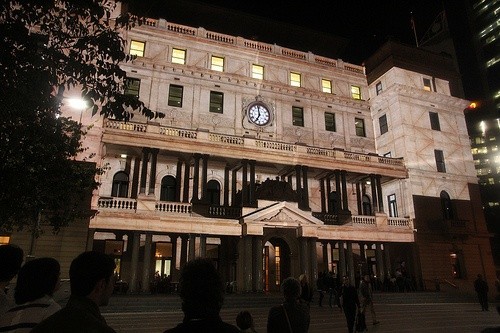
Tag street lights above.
[73,98,87,160]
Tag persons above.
[0,244,24,310]
[156,273,170,293]
[236,311,258,333]
[266,277,311,333]
[0,257,63,333]
[28,251,117,333]
[297,261,412,308]
[342,277,361,333]
[494,278,500,315]
[474,273,489,312]
[164,259,241,333]
[359,274,380,326]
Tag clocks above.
[247,101,273,128]
